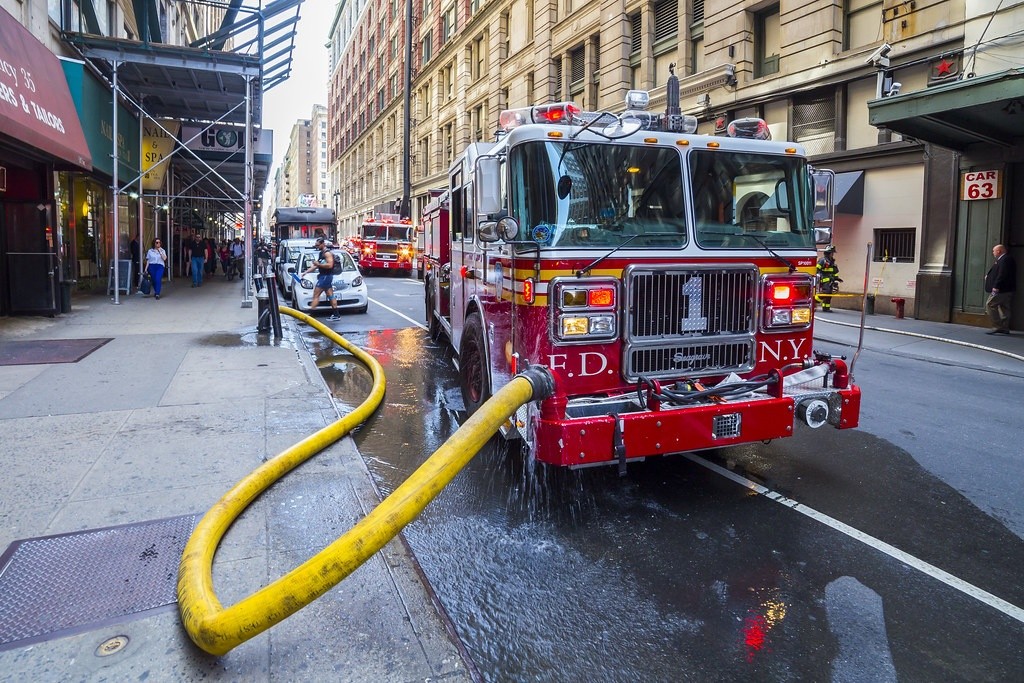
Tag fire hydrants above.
[255,288,271,334]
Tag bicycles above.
[226,256,238,281]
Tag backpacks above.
[322,249,342,275]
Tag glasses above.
[155,241,163,245]
[316,242,322,246]
[196,237,200,238]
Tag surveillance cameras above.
[865,44,891,65]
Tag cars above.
[288,249,369,315]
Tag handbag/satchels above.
[140,274,151,295]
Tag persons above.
[130,234,139,288]
[189,233,208,288]
[317,229,327,239]
[211,239,218,268]
[184,239,192,278]
[814,244,843,313]
[985,245,1016,335]
[203,238,212,278]
[217,238,244,278]
[301,237,340,321]
[144,238,167,300]
[254,236,271,279]
[341,242,350,269]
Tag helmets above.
[824,246,837,255]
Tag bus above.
[270,207,339,266]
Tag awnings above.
[0,5,92,171]
[760,171,865,216]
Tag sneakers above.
[326,315,341,321]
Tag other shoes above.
[155,294,159,300]
[986,327,1010,335]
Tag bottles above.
[319,259,327,264]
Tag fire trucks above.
[423,61,863,477]
[356,212,414,278]
[417,226,425,279]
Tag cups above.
[892,257,896,263]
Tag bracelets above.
[318,265,320,267]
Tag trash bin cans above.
[60,278,76,314]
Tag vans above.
[279,238,334,298]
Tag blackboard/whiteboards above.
[108,260,133,290]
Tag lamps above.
[697,95,709,105]
